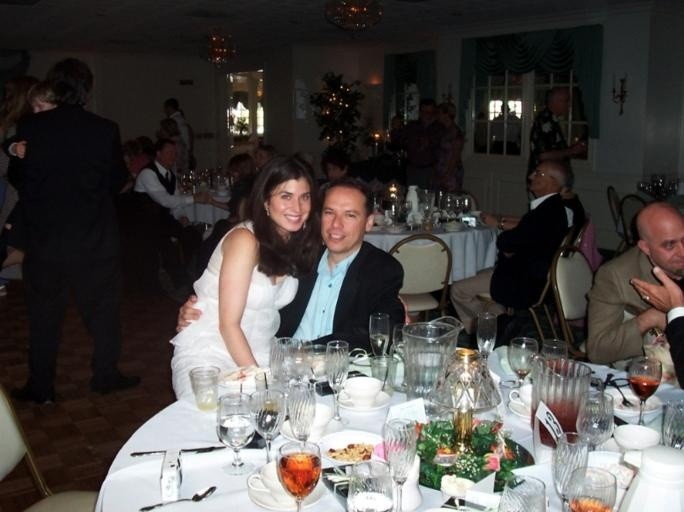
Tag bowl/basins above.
[337,391,392,431]
[341,376,384,407]
[507,383,533,412]
[612,424,661,451]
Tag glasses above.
[534,169,556,179]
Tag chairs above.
[144,167,683,358]
[0,384,97,511]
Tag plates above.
[246,487,327,510]
[320,430,385,466]
[508,401,533,425]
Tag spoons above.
[138,485,217,512]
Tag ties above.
[164,171,170,183]
[555,119,567,142]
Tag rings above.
[642,293,652,304]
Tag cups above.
[403,316,466,401]
[367,313,391,357]
[427,350,502,412]
[561,466,617,511]
[532,358,594,449]
[542,339,570,374]
[575,392,616,452]
[302,343,328,383]
[369,451,422,510]
[590,385,662,417]
[497,472,548,511]
[254,373,276,393]
[346,458,394,511]
[661,399,684,449]
[248,461,298,506]
[188,365,221,412]
[550,431,590,501]
[371,356,400,397]
[268,338,310,391]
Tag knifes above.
[128,444,227,456]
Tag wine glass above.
[324,340,349,422]
[175,163,236,204]
[508,338,538,397]
[381,417,415,511]
[277,441,322,510]
[475,312,498,360]
[392,322,407,387]
[367,178,479,236]
[627,356,662,424]
[217,393,258,476]
[249,389,286,472]
[279,383,321,457]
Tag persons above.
[448,161,586,336]
[0,56,135,405]
[120,97,277,290]
[626,265,683,392]
[171,176,407,360]
[582,201,683,387]
[323,152,350,184]
[525,87,586,191]
[410,97,465,194]
[165,153,412,409]
